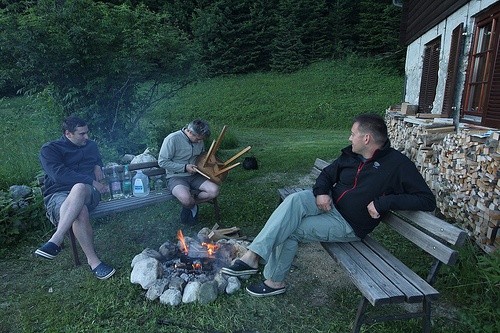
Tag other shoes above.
[189,205,198,226]
[180,207,190,224]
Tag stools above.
[194,124,252,184]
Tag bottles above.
[154,177,164,194]
[132,170,150,197]
[111,166,123,199]
[122,165,133,197]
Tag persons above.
[158,119,220,226]
[35,116,116,280]
[222,113,437,297]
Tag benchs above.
[39,161,220,265]
[277,158,466,333]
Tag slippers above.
[245,281,286,297]
[221,259,259,275]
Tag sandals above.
[35,242,61,260]
[90,260,116,280]
